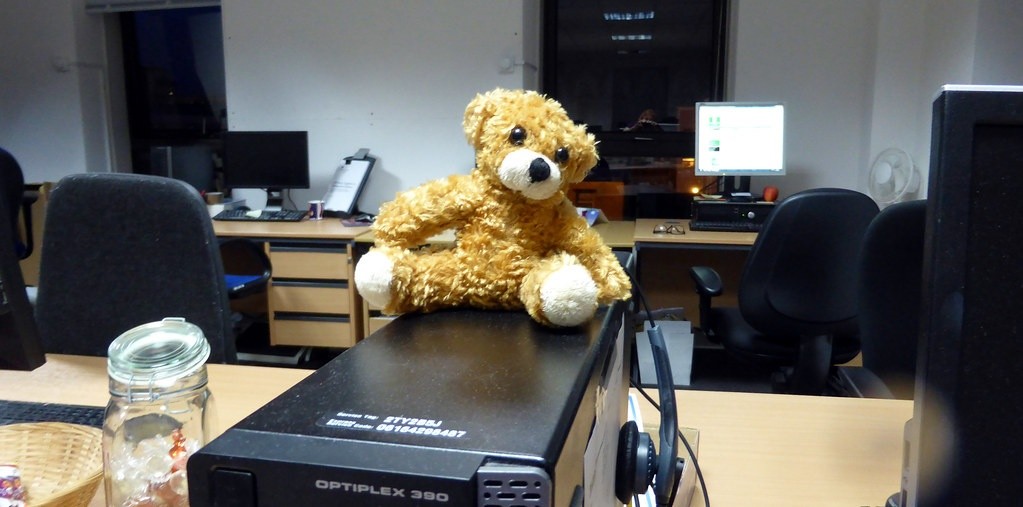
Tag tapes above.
[205,192,223,204]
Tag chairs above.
[835,198,930,399]
[37,170,237,366]
[686,187,883,387]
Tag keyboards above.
[212,210,308,222]
[0,400,106,429]
[690,223,762,232]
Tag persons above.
[629,108,659,133]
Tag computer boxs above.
[692,203,777,222]
[186,252,636,507]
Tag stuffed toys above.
[353,85,633,328]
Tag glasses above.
[653,225,685,234]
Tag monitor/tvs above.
[694,101,784,201]
[217,131,309,210]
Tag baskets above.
[0,422,102,507]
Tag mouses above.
[355,213,375,223]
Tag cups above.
[207,193,223,205]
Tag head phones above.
[616,322,710,506]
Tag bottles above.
[101,315,212,507]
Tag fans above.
[866,145,921,208]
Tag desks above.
[633,213,761,252]
[207,215,372,353]
[0,350,911,507]
[355,221,637,342]
